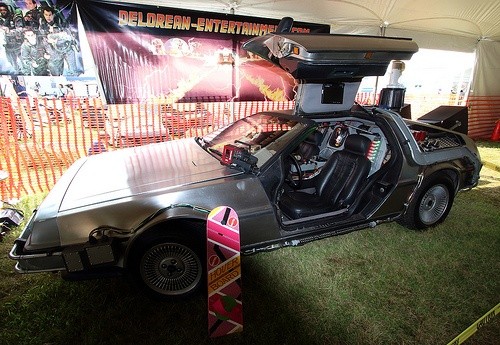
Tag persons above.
[0,0,82,75]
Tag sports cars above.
[9,17,483,304]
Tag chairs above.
[296,132,325,162]
[278,134,371,220]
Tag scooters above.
[208,206,243,345]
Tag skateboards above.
[206,207,242,340]
